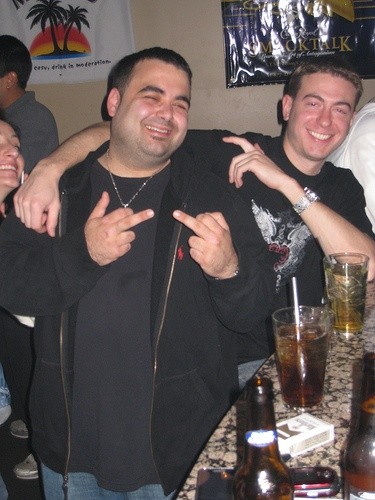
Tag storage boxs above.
[276,413,334,458]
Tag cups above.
[320,252,369,332]
[271,305,333,408]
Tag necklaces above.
[105,148,166,209]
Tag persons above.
[0,46,277,499]
[0,35,59,480]
[14,49,375,500]
[325,94,375,236]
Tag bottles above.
[340,353,374,500]
[233,378,295,500]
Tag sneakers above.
[9,416,30,438]
[12,453,41,480]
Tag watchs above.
[292,186,321,217]
